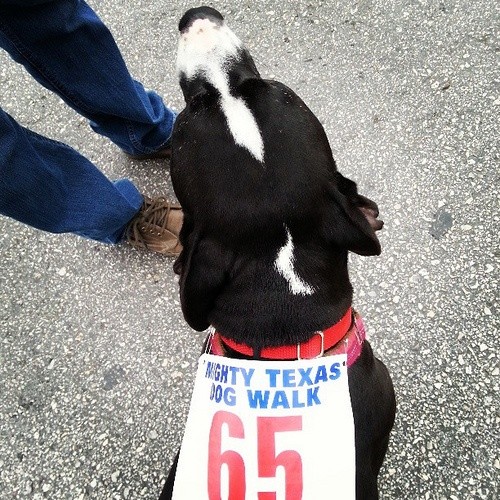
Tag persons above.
[0,1,188,257]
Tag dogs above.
[158,4,395,500]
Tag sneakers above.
[123,137,171,160]
[121,192,184,257]
